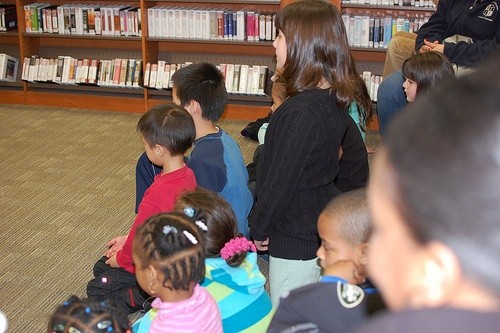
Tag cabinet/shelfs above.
[0,0,440,132]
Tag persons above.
[267,63,500,333]
[247,0,374,313]
[401,49,456,103]
[48,62,287,333]
[377,0,500,138]
[382,0,438,80]
[315,186,378,296]
[264,73,375,184]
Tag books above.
[340,0,436,49]
[0,0,18,33]
[21,55,142,89]
[0,54,19,82]
[358,71,384,102]
[147,4,280,43]
[144,61,272,97]
[24,3,142,38]
